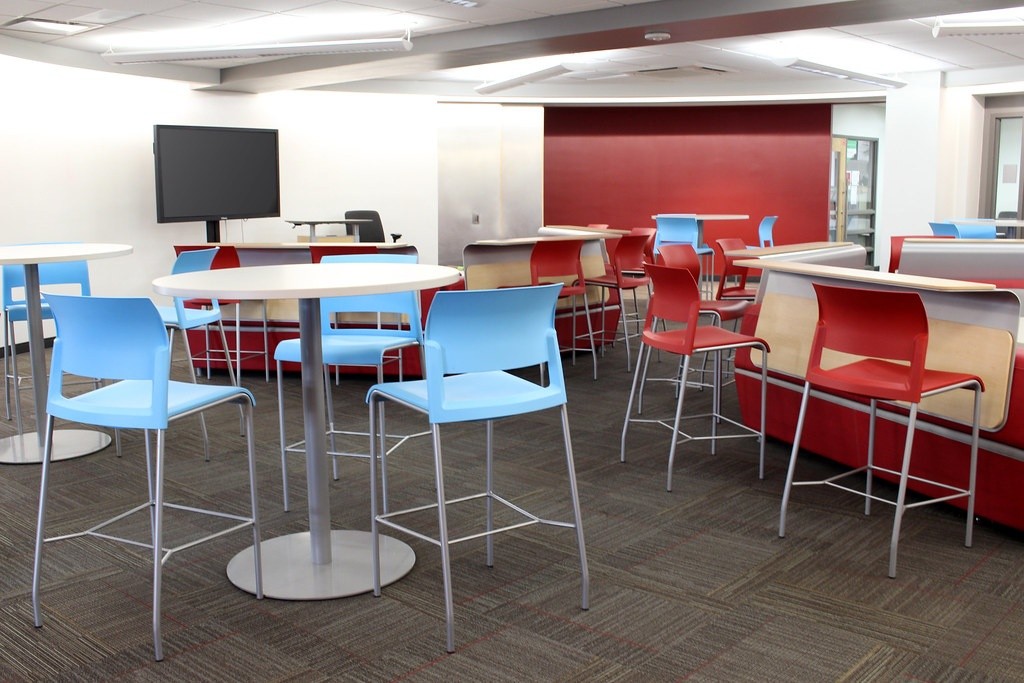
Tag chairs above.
[653,217,715,302]
[274,255,426,519]
[620,260,772,491]
[929,222,961,239]
[587,224,609,229]
[2,242,107,436]
[32,292,264,662]
[498,240,598,387]
[724,216,778,289]
[656,243,751,399]
[887,236,955,274]
[309,246,402,385]
[345,210,385,242]
[366,282,592,654]
[572,235,662,373]
[700,237,762,391]
[609,227,668,348]
[115,246,245,461]
[778,283,985,579]
[946,219,996,239]
[173,246,270,383]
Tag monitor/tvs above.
[153,124,281,223]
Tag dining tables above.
[957,220,1024,226]
[651,215,750,301]
[0,243,133,465]
[150,262,460,601]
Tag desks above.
[898,238,1024,343]
[724,241,866,271]
[173,241,441,377]
[442,226,631,364]
[732,260,1023,530]
